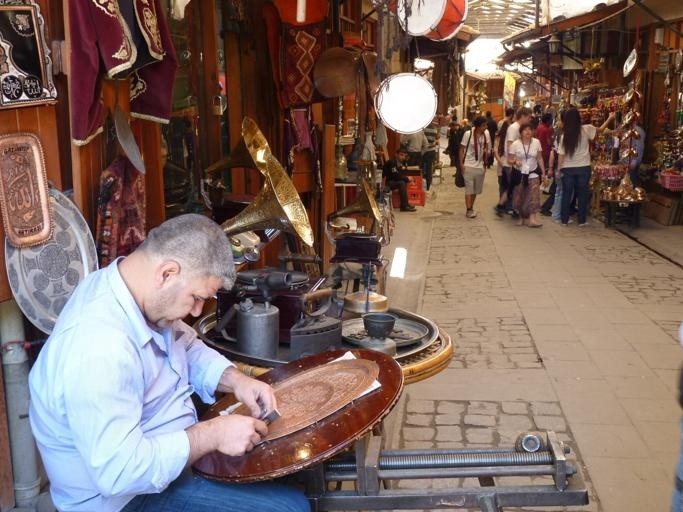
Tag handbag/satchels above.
[455,165,464,187]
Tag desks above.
[189,300,452,401]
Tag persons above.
[670,321,683,512]
[27,214,312,512]
[619,117,647,187]
[442,103,617,228]
[380,148,416,212]
[398,129,430,166]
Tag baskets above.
[660,175,683,189]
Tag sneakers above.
[466,209,477,218]
[494,205,543,227]
[401,205,417,211]
[540,206,590,227]
